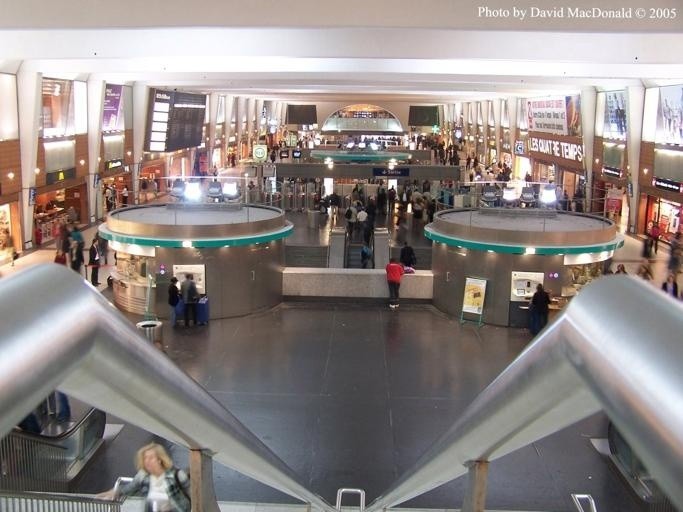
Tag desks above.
[174,296,209,325]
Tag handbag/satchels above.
[176,300,184,316]
[345,210,352,219]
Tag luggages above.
[198,296,208,325]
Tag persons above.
[3,134,682,437]
[96,443,191,512]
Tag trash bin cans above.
[308,210,320,229]
[136,321,162,343]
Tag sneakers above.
[389,304,399,309]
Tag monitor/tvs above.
[517,289,525,294]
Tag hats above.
[170,277,178,282]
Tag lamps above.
[481,183,556,206]
[28,187,37,207]
[279,150,301,158]
[93,174,100,188]
[628,184,633,196]
[346,142,378,152]
